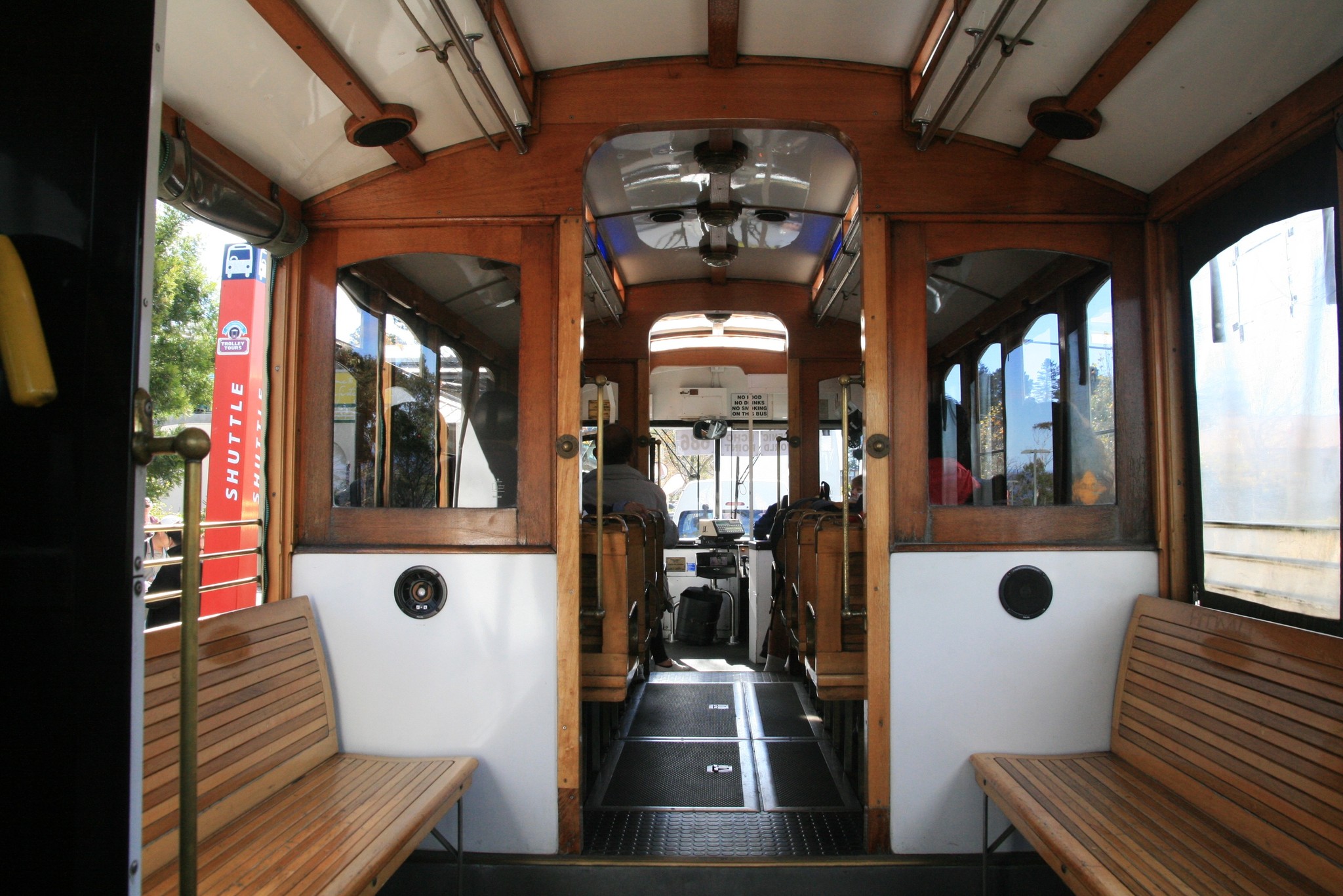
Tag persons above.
[145,498,159,540]
[582,424,679,549]
[754,503,777,541]
[1068,404,1114,503]
[930,396,981,505]
[583,501,652,524]
[763,589,790,672]
[849,474,862,500]
[145,521,205,629]
[144,523,169,587]
[199,533,205,555]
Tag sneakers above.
[654,658,690,672]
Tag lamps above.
[693,139,749,175]
[705,313,731,323]
[699,244,739,268]
[697,200,742,228]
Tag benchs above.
[141,594,477,895]
[767,508,863,799]
[973,595,1343,896]
[579,509,666,802]
